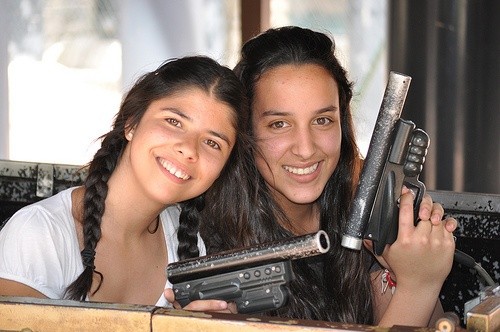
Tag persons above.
[0,55,248,314]
[203,26,457,328]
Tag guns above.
[341,70,430,256]
[168,229,331,314]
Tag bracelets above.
[378,268,396,296]
